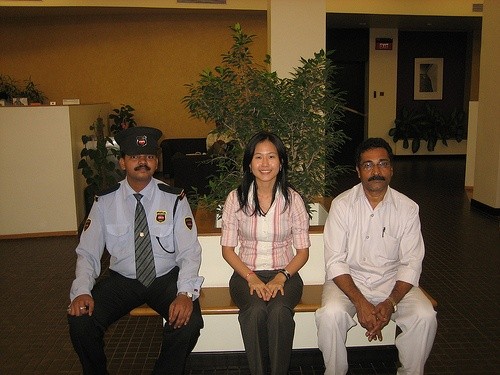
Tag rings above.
[67,305,72,313]
[79,306,86,310]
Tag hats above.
[114,126,163,156]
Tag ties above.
[133,193,156,288]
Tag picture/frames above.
[415,58,443,100]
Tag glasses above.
[361,162,390,172]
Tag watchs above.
[277,267,291,280]
[387,296,398,314]
[178,292,194,302]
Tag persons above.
[319,136,438,375]
[68,126,205,375]
[220,131,310,374]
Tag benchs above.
[130,284,438,352]
